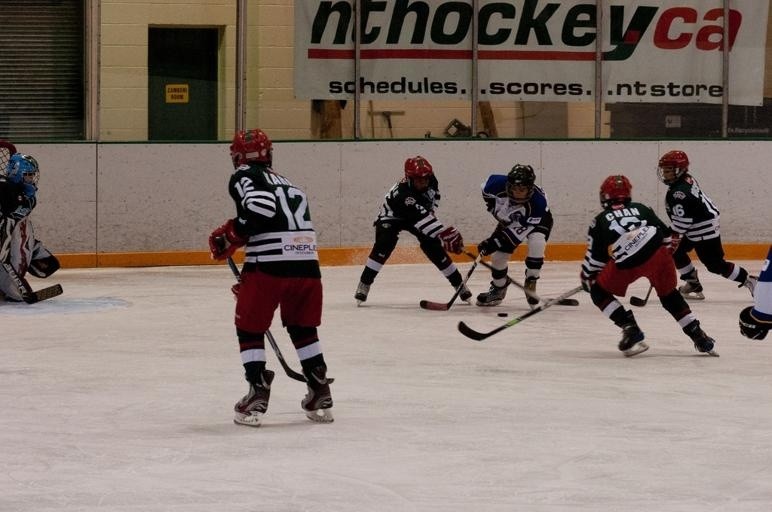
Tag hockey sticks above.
[221,239,335,384]
[459,285,584,341]
[4,260,64,304]
[630,285,652,307]
[460,250,580,306]
[420,222,502,311]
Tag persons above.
[210,129,334,415]
[0,152,58,303]
[476,164,553,305]
[580,173,715,354]
[738,245,772,340]
[354,154,473,301]
[656,150,761,295]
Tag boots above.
[523,275,541,311]
[615,310,650,357]
[232,369,275,427]
[454,282,473,306]
[738,272,758,297]
[685,320,721,358]
[354,278,371,307]
[677,268,705,301]
[476,277,513,308]
[300,365,336,422]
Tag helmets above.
[6,153,41,188]
[506,164,538,203]
[598,174,632,208]
[405,155,433,191]
[657,150,690,185]
[228,129,274,171]
